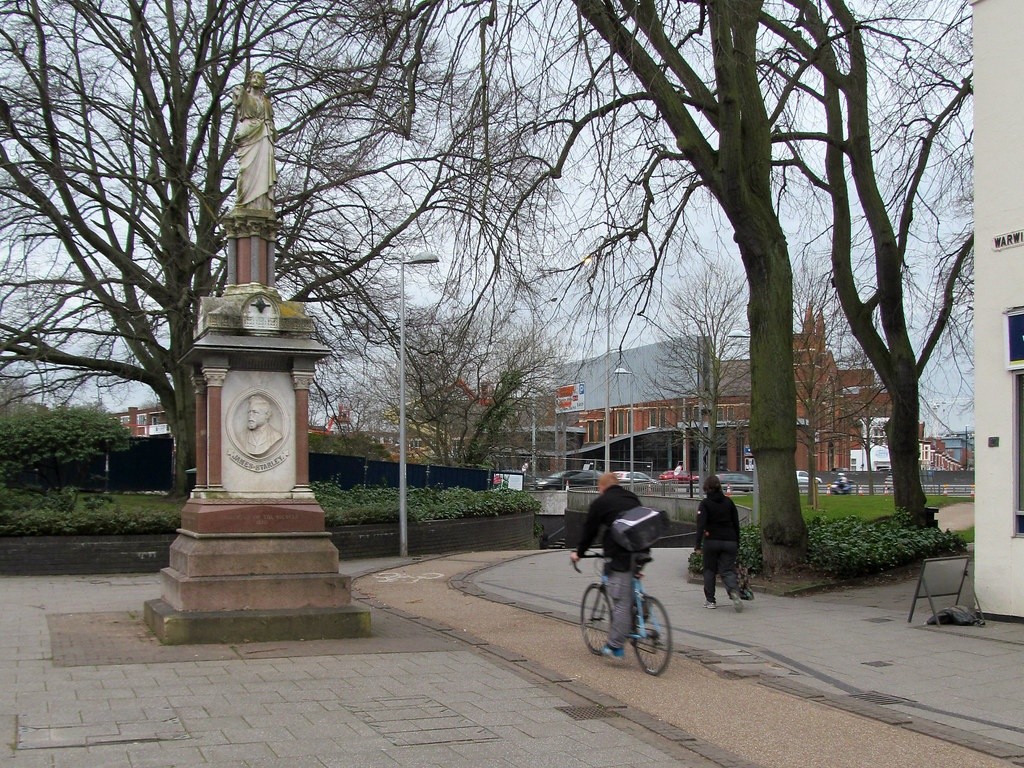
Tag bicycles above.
[569,549,672,676]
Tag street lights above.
[508,297,558,480]
[400,251,440,559]
[613,367,635,493]
[965,425,970,470]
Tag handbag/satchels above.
[612,506,670,551]
[727,567,754,600]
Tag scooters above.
[829,479,854,495]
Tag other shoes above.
[635,605,648,626]
[600,646,624,658]
[703,601,716,608]
[729,597,743,613]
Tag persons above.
[694,475,744,612]
[231,71,279,214]
[834,472,847,493]
[570,475,649,659]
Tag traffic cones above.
[826,486,831,496]
[969,487,974,496]
[725,484,731,497]
[884,486,889,495]
[943,486,947,496]
[858,485,864,496]
[565,480,569,491]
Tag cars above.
[658,471,699,483]
[613,471,657,484]
[715,474,753,492]
[534,469,603,490]
[795,471,822,485]
